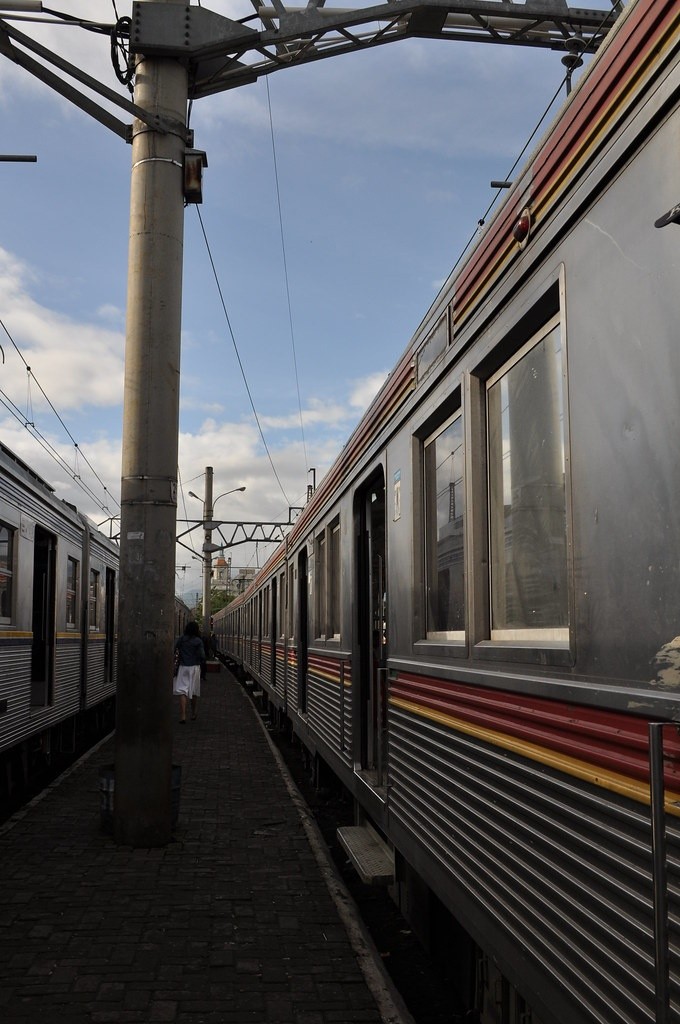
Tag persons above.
[172,622,204,724]
[209,631,220,660]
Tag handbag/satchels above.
[173,636,183,677]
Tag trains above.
[0,437,195,816]
[210,1,680,1024]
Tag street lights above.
[187,466,245,656]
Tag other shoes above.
[179,716,186,724]
[191,713,200,720]
